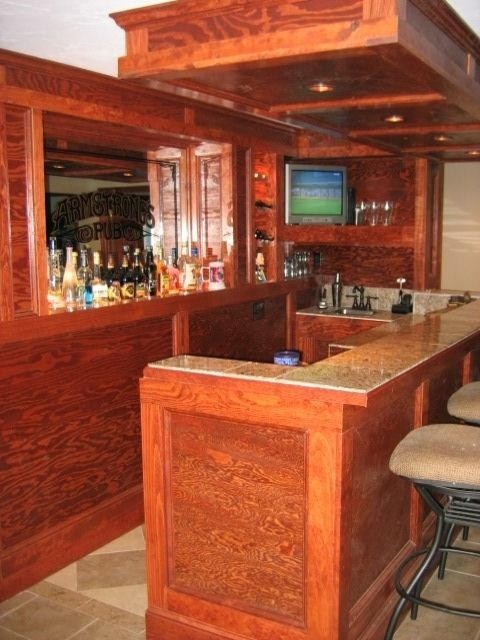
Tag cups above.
[355,198,395,228]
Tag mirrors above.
[31,107,247,316]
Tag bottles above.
[332,270,342,309]
[254,252,267,283]
[284,250,310,280]
[45,234,235,314]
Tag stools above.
[437,380,480,581]
[383,423,480,640]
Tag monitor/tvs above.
[285,164,348,224]
